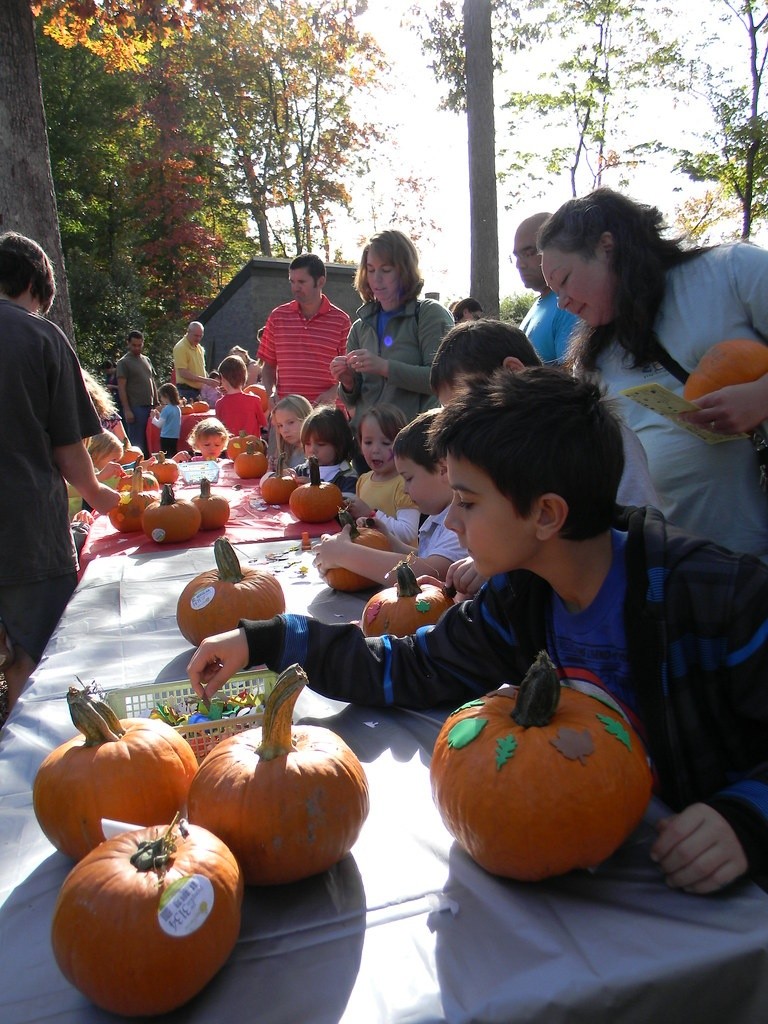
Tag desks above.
[0,406,768,1024]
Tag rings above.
[361,362,362,367]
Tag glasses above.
[471,311,479,319]
[510,251,537,263]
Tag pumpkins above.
[176,537,287,648]
[243,384,269,413]
[184,664,370,885]
[430,648,653,880]
[49,812,245,1016]
[32,686,199,861]
[317,508,394,590]
[683,340,768,401]
[109,436,229,545]
[361,561,455,638]
[178,395,210,414]
[226,431,343,523]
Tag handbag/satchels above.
[201,382,223,409]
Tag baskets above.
[106,668,279,766]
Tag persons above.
[150,383,181,460]
[202,371,224,409]
[448,299,483,330]
[172,418,229,463]
[101,360,125,427]
[214,355,266,459]
[0,233,121,731]
[257,326,267,343]
[116,330,161,461]
[282,406,358,501]
[227,346,263,389]
[430,319,671,603]
[270,395,313,477]
[61,367,131,555]
[256,253,352,473]
[187,367,768,894]
[312,408,471,593]
[330,229,455,477]
[537,190,768,577]
[513,213,585,378]
[173,321,221,405]
[342,403,421,556]
